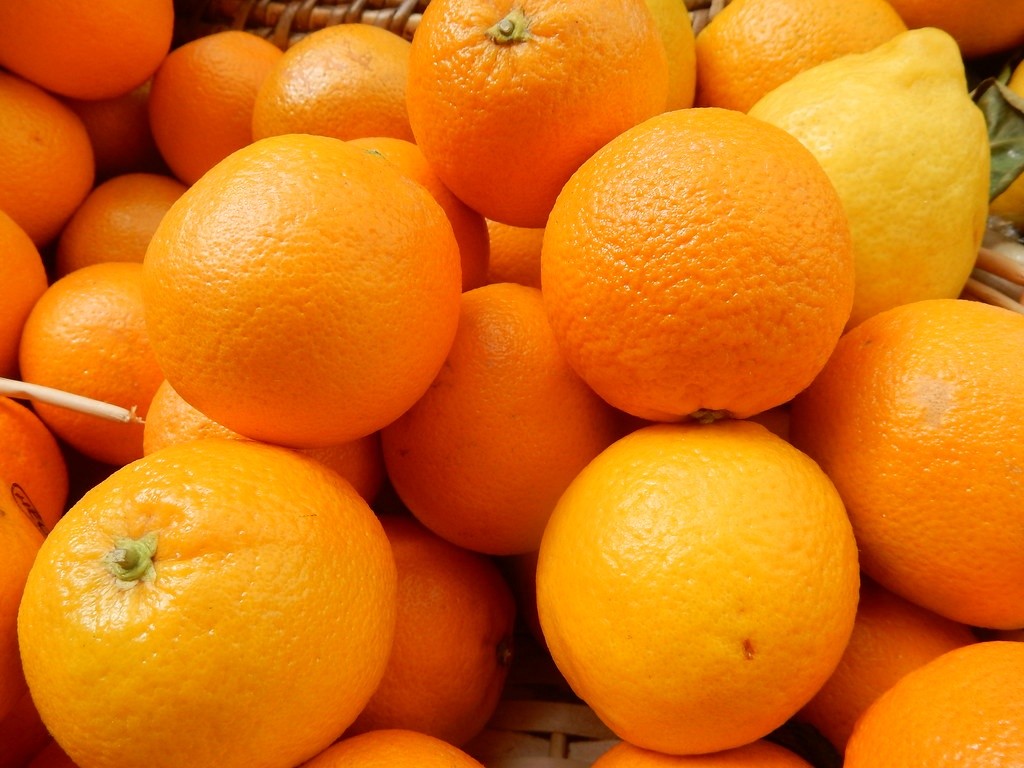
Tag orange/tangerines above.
[0,0,1024,768]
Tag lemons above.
[744,26,992,323]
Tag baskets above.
[0,0,1024,768]
